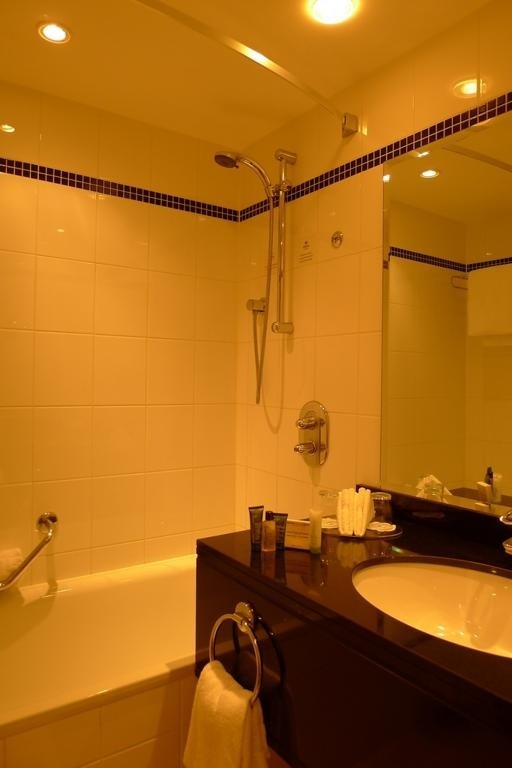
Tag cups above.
[318,490,339,527]
[423,483,444,500]
[368,492,391,531]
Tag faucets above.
[500,509,512,555]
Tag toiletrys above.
[260,510,276,553]
[308,505,322,554]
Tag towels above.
[183,660,272,768]
[417,474,453,497]
[338,487,376,536]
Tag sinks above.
[353,557,512,660]
